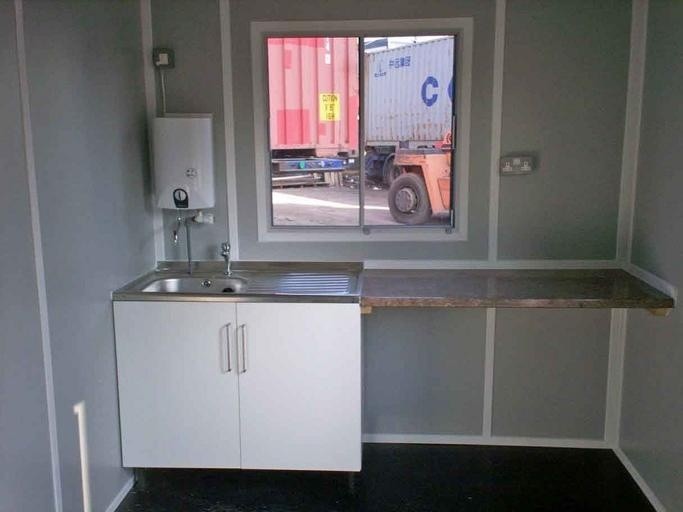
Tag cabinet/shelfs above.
[113,299,364,475]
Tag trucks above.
[362,36,453,188]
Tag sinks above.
[141,275,246,295]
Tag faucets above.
[172,219,181,243]
[219,242,232,275]
